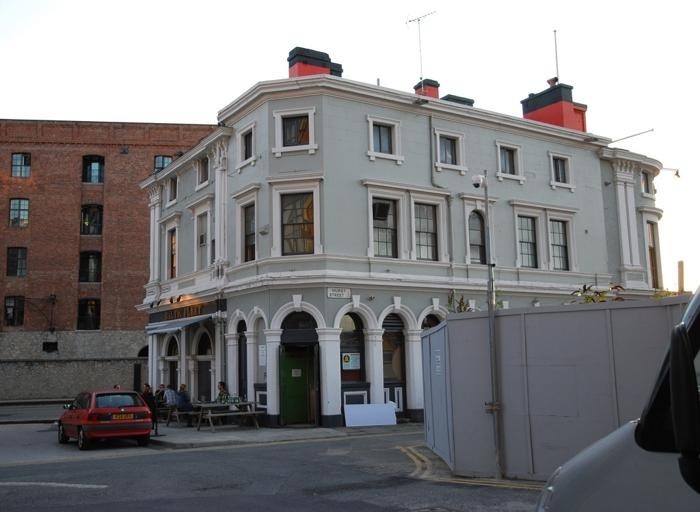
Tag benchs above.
[170,411,266,430]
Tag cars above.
[58,388,152,450]
[536,286,700,512]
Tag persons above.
[216,381,230,404]
[114,384,120,391]
[141,383,194,426]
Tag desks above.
[192,402,259,432]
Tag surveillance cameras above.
[472,174,482,188]
